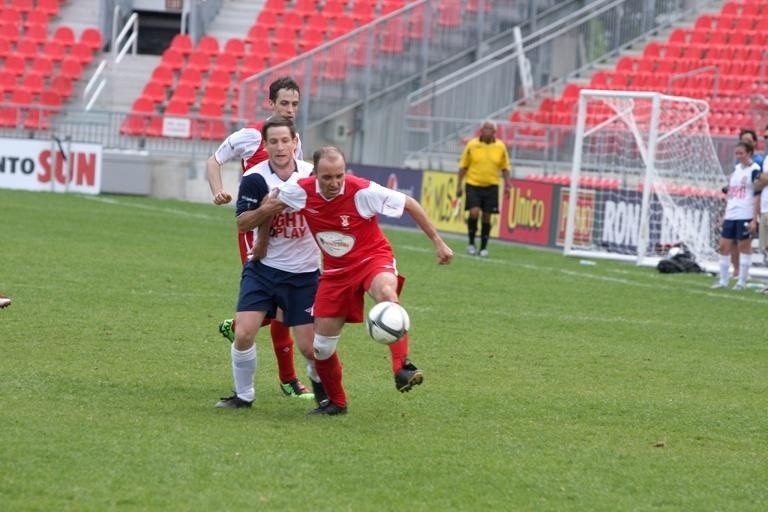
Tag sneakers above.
[214,394,252,410]
[307,400,347,416]
[218,319,234,343]
[279,378,316,400]
[304,364,332,399]
[394,359,424,394]
[711,274,768,294]
[466,245,488,257]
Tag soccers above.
[366,301,410,344]
[668,246,683,257]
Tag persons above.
[206,75,316,401]
[247,146,453,417]
[214,115,331,410]
[456,119,512,259]
[710,125,768,294]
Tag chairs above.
[0,1,104,128]
[248,0,405,49]
[118,34,312,139]
[512,1,768,148]
